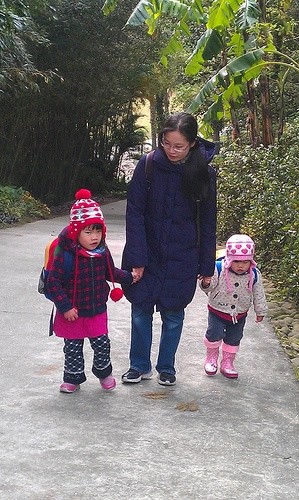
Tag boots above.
[220,342,240,378]
[203,335,222,376]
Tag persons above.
[120,113,217,385]
[198,234,268,378]
[46,189,139,393]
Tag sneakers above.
[121,368,153,383]
[59,382,80,393]
[99,374,116,391]
[157,372,176,386]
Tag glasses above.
[160,140,192,153]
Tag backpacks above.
[38,236,74,337]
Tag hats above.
[224,234,257,293]
[69,189,123,313]
